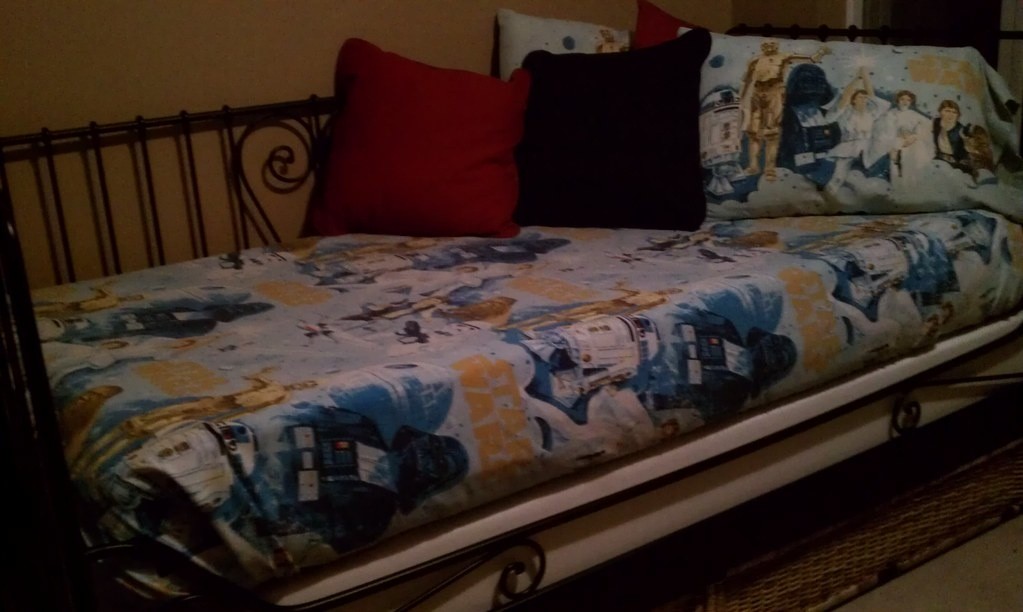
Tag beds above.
[0,22,1023,612]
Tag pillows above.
[678,27,1023,224]
[302,35,525,239]
[511,26,712,231]
[495,8,629,78]
[633,2,694,50]
